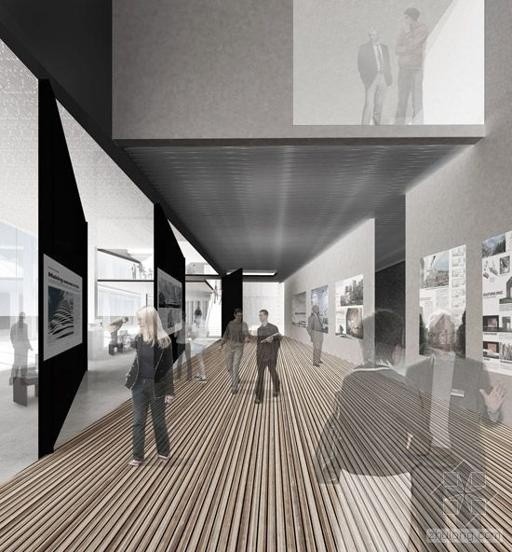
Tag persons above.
[393,8,429,124]
[110,316,129,344]
[124,304,175,468]
[253,309,284,404]
[8,312,34,385]
[173,311,193,382]
[335,308,432,552]
[304,305,325,367]
[192,309,210,382]
[357,29,393,125]
[218,307,251,395]
[406,312,507,552]
